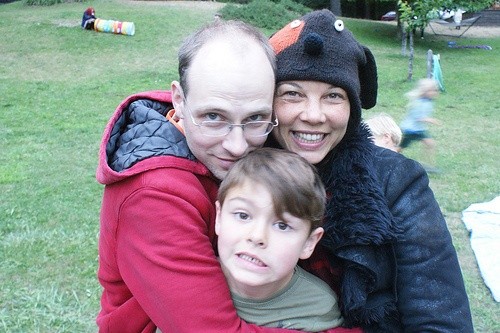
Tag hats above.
[268,9,378,129]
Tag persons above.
[157,147,343,333]
[82,8,95,29]
[363,113,402,152]
[399,80,444,174]
[97,22,362,333]
[268,9,474,333]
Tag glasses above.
[183,96,279,137]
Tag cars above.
[381,11,397,21]
